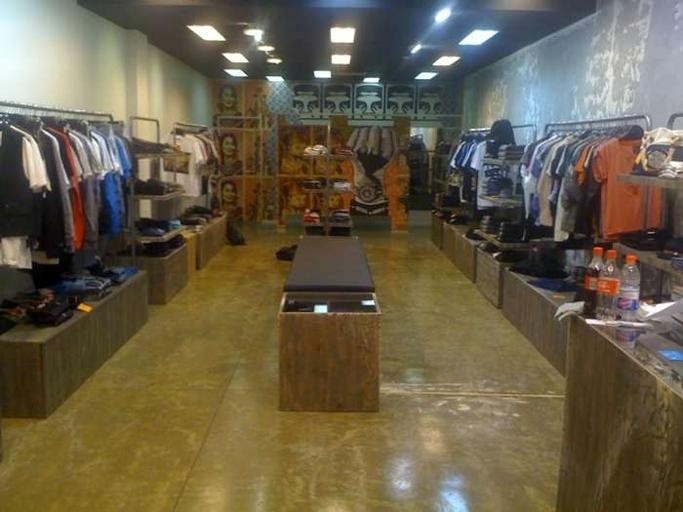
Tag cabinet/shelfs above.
[613,112,683,305]
[301,153,354,229]
[130,117,189,269]
[431,154,465,214]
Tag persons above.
[213,84,243,129]
[217,132,242,176]
[219,180,243,222]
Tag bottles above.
[612,324,638,357]
[578,244,608,321]
[614,253,644,322]
[594,249,620,322]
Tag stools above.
[285,235,374,292]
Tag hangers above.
[1,101,116,135]
[170,122,209,137]
[544,116,644,141]
